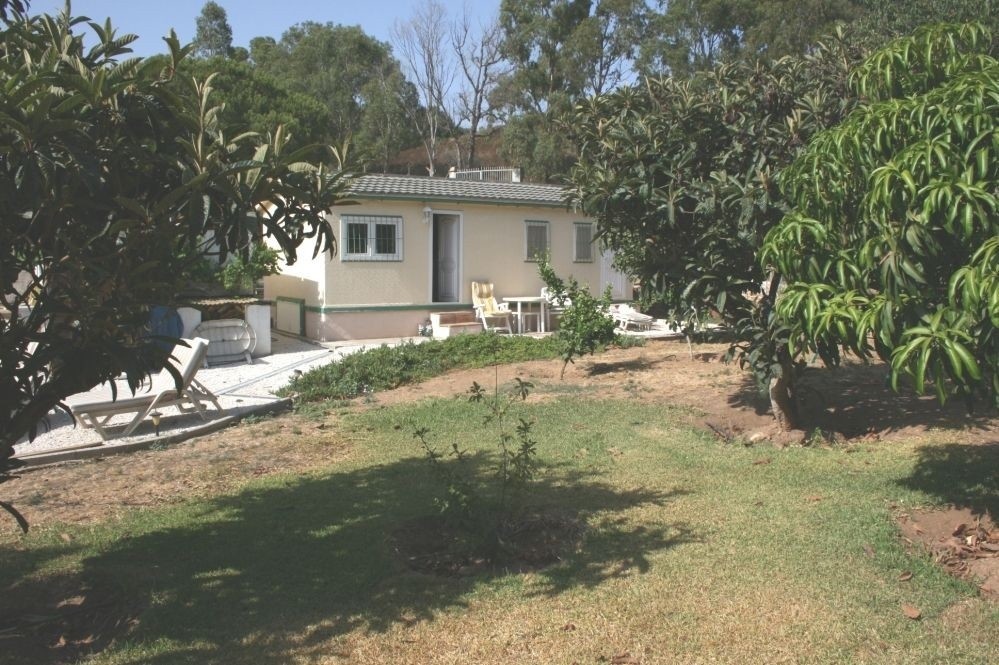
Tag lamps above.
[422,201,432,224]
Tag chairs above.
[541,286,573,332]
[470,281,514,338]
[68,337,226,442]
[600,304,654,331]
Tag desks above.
[191,318,257,369]
[504,296,549,334]
